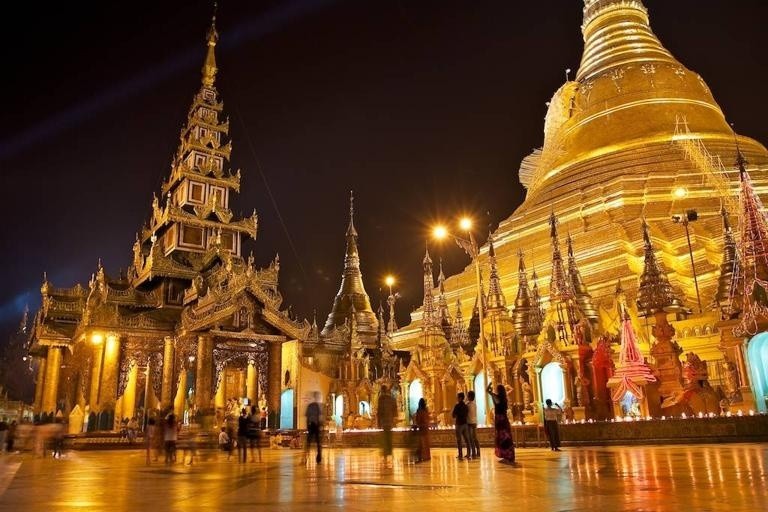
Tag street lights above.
[385,276,397,334]
[670,185,706,313]
[432,217,491,427]
[91,333,117,408]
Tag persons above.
[5,420,16,451]
[259,406,270,430]
[218,426,232,450]
[246,405,262,464]
[451,391,471,459]
[117,408,218,467]
[484,381,516,465]
[236,407,252,464]
[415,397,432,465]
[542,398,563,453]
[298,390,323,468]
[376,383,398,464]
[0,416,7,452]
[465,390,481,459]
